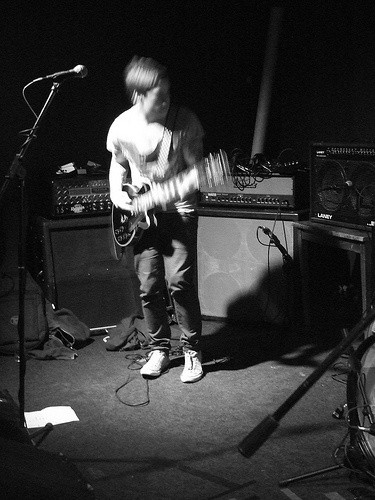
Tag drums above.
[345,334,375,488]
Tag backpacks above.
[0,267,46,356]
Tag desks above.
[293,222,375,340]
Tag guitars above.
[110,148,227,249]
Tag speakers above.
[28,214,146,336]
[308,141,375,231]
[192,207,307,322]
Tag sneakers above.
[180,350,203,382]
[140,350,170,377]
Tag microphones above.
[262,227,292,262]
[33,64,88,80]
[330,180,352,189]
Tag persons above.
[105,56,208,384]
